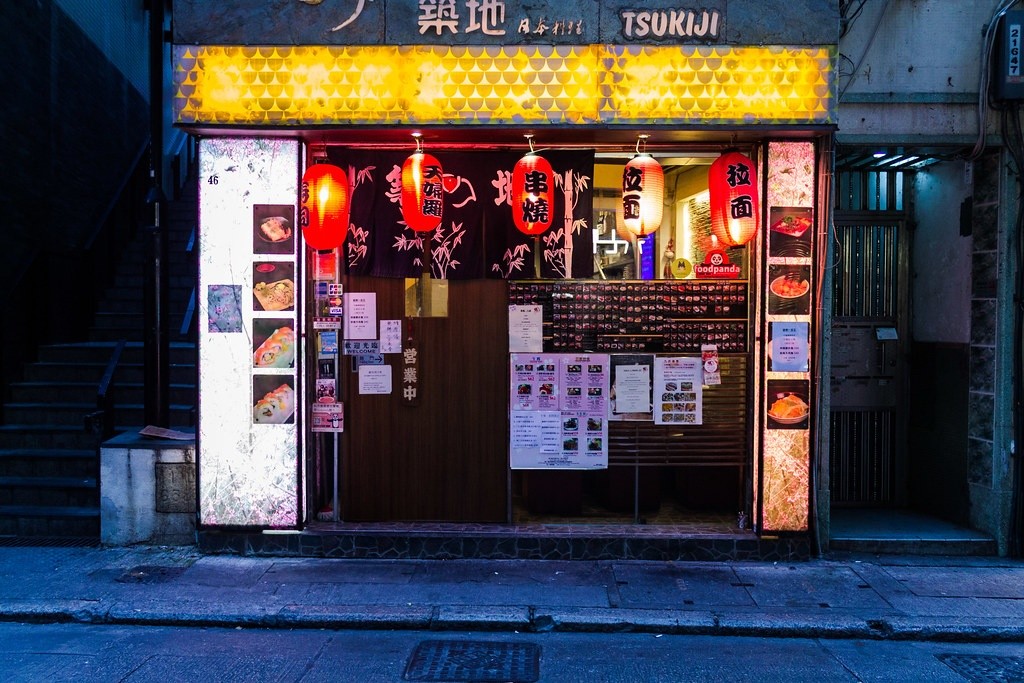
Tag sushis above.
[254,384,293,422]
[254,327,293,366]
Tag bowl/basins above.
[768,391,809,424]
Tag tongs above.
[253,279,294,311]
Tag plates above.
[253,328,294,368]
[768,339,809,361]
[771,215,811,237]
[256,264,275,273]
[257,216,291,243]
[564,428,577,430]
[770,274,810,298]
[253,383,294,424]
